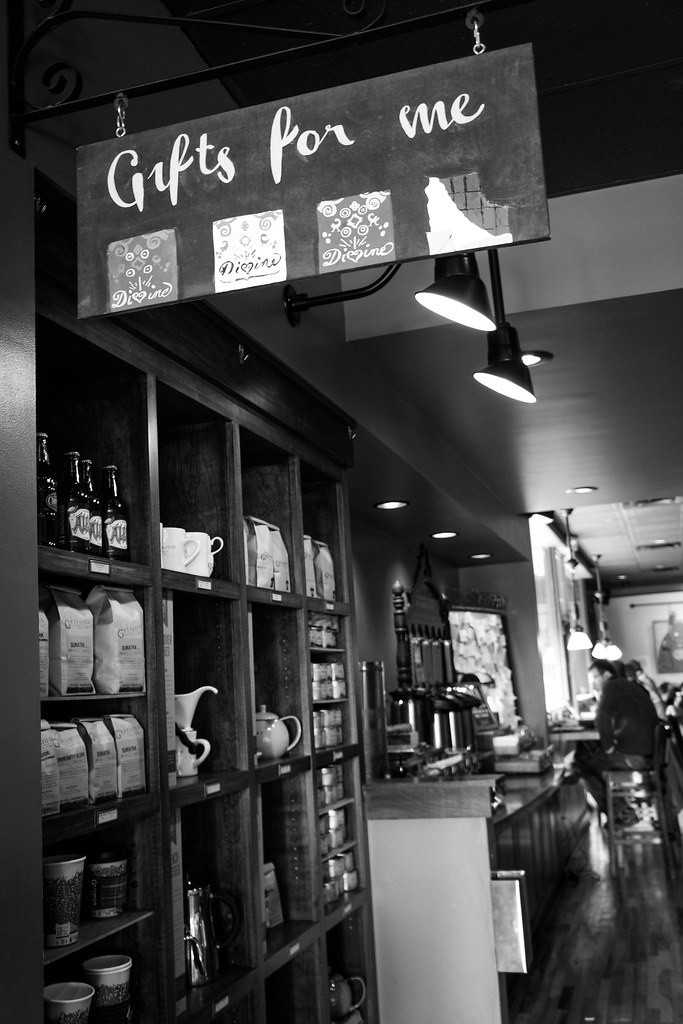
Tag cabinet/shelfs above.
[34,283,380,1024]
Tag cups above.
[86,860,128,918]
[43,854,87,946]
[159,523,224,577]
[176,731,210,777]
[84,955,132,1005]
[44,982,95,1024]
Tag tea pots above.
[327,966,366,1020]
[256,705,301,761]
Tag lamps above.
[281,245,538,405]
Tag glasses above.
[588,673,600,681]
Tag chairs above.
[599,720,673,845]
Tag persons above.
[568,658,669,828]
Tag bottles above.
[37,432,130,560]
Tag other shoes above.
[603,808,639,832]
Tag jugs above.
[184,884,238,987]
